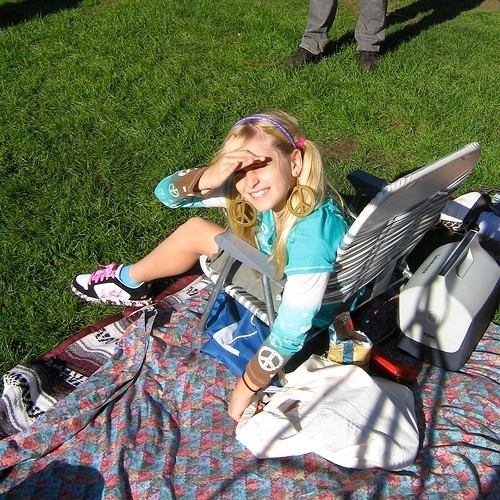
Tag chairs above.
[193,140,482,391]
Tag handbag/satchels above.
[234,355,417,469]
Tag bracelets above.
[242,372,258,393]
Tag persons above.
[284,0,388,74]
[69,109,366,424]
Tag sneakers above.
[72,263,153,308]
